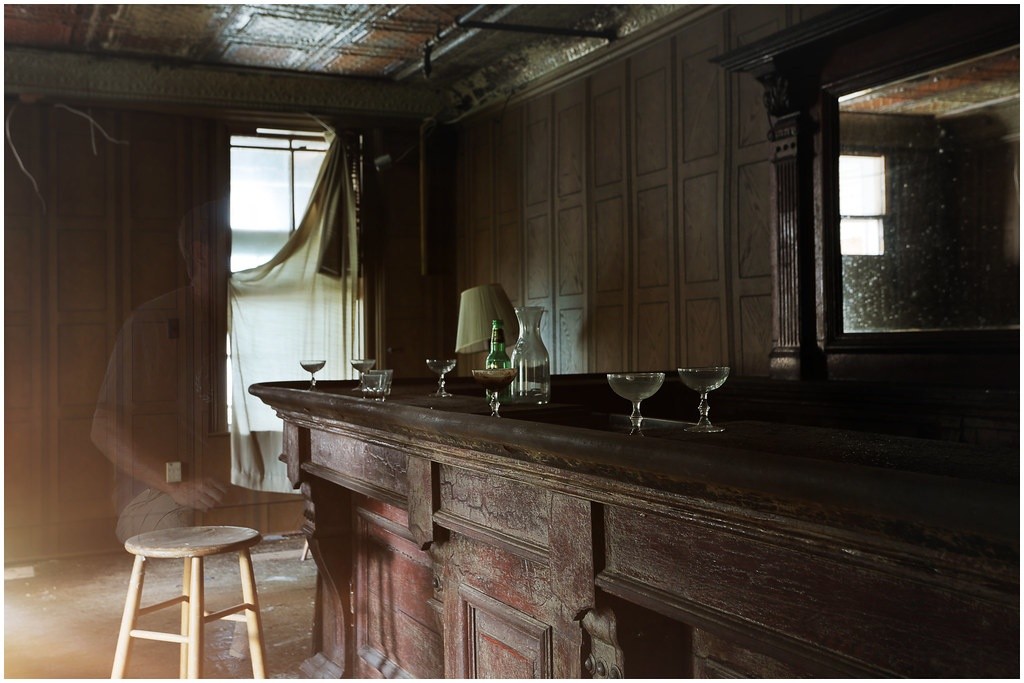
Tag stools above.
[109,524,268,680]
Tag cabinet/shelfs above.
[247,379,1017,679]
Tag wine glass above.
[425,358,457,398]
[606,372,666,438]
[299,359,326,393]
[350,358,375,392]
[677,366,730,434]
[471,368,518,418]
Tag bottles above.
[485,319,517,406]
[510,306,551,406]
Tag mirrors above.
[820,23,1020,353]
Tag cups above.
[360,373,387,405]
[369,369,393,396]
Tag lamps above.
[454,282,521,396]
